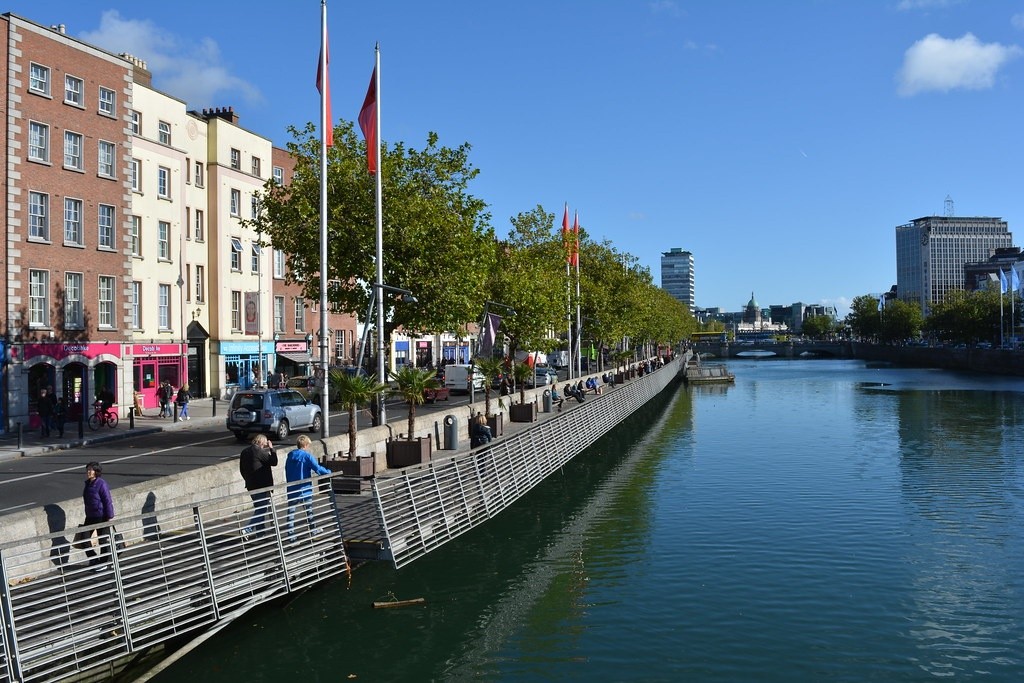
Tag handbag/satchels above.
[113,526,126,551]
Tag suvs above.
[329,365,369,383]
[226,389,322,442]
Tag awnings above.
[277,352,320,366]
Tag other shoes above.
[159,413,162,418]
[187,416,190,420]
[290,536,297,542]
[163,415,166,418]
[73,524,85,549]
[313,529,323,536]
[95,565,108,571]
[241,528,249,541]
[179,417,183,421]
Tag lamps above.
[192,307,202,319]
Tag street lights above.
[475,301,517,357]
[353,283,418,382]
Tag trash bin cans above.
[444,415,458,450]
[542,389,552,413]
[608,372,614,382]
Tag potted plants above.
[383,366,439,468]
[314,369,382,488]
[607,350,636,384]
[467,354,507,437]
[504,361,538,422]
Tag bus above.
[691,332,725,342]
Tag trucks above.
[445,364,486,394]
[548,351,568,369]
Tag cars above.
[487,369,506,388]
[528,363,559,389]
[422,372,449,405]
[286,375,342,406]
[574,356,592,371]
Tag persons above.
[246,301,255,323]
[39,385,66,439]
[586,371,615,395]
[239,435,278,541]
[82,461,116,572]
[498,377,509,396]
[441,357,475,369]
[470,415,492,485]
[552,380,586,408]
[285,434,331,541]
[153,380,194,422]
[94,385,115,427]
[266,370,290,389]
[636,353,675,378]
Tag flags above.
[562,210,578,266]
[878,295,886,311]
[1000,268,1008,293]
[315,23,333,148]
[1012,266,1019,291]
[358,67,377,173]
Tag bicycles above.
[88,404,119,432]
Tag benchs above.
[551,381,608,405]
